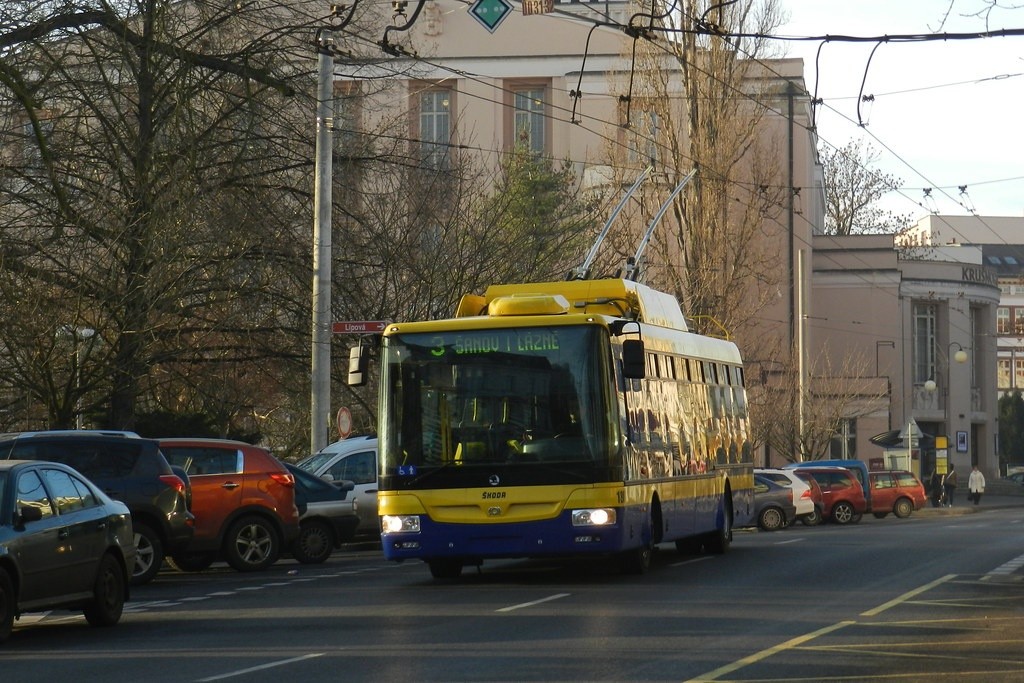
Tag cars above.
[754,475,797,531]
[0,459,136,643]
[277,459,358,567]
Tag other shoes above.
[948,505,952,508]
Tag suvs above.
[153,439,300,574]
[293,434,383,551]
[778,467,867,524]
[753,467,814,529]
[868,469,927,518]
[0,430,195,590]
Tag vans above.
[781,460,874,523]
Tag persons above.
[453,406,522,464]
[968,465,985,505]
[941,463,957,508]
[930,465,941,506]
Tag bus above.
[348,279,756,582]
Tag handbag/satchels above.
[967,489,972,500]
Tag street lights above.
[55,325,95,432]
[924,342,968,491]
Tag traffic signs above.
[332,320,395,333]
